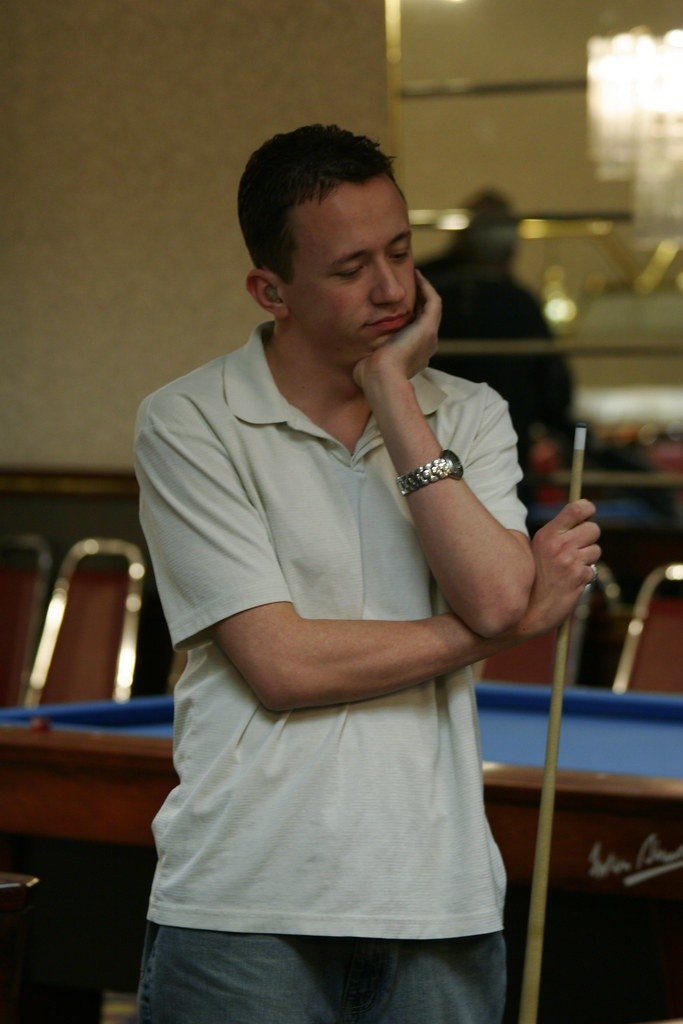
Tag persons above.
[134,126,602,1024]
[418,186,576,489]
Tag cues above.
[516,424,589,1024]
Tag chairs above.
[22,534,151,713]
[470,562,617,684]
[610,563,683,693]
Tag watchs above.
[396,448,465,496]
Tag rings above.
[591,564,597,585]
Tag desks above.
[0,681,683,905]
